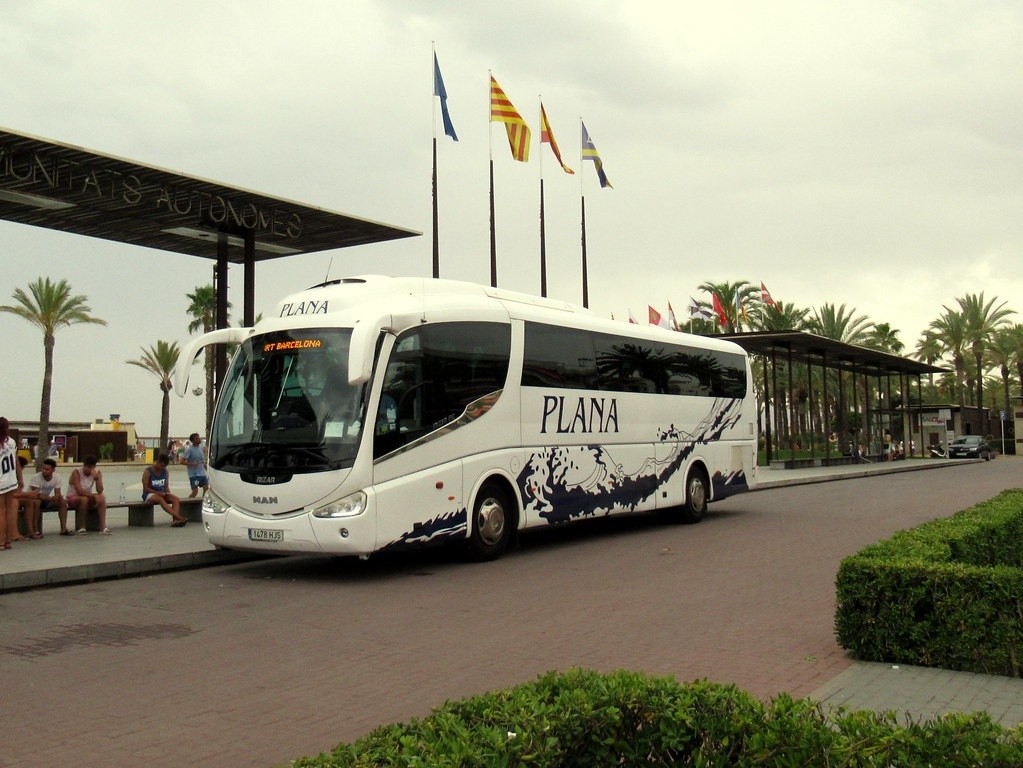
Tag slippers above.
[76,528,87,535]
[8,536,30,541]
[99,528,114,535]
[60,528,75,536]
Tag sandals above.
[1,541,12,551]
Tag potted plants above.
[100,443,113,463]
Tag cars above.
[948,435,996,461]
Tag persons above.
[0,416,31,552]
[829,432,835,451]
[180,433,208,498]
[65,455,112,536]
[27,458,74,539]
[141,452,188,528]
[167,436,185,465]
[336,365,396,420]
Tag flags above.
[491,74,531,162]
[737,290,747,323]
[668,303,679,332]
[649,306,661,326]
[713,293,726,327]
[762,283,782,317]
[434,49,460,142]
[582,120,615,191]
[691,306,713,319]
[540,101,575,175]
[629,318,634,323]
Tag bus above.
[175,273,757,558]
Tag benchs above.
[770,458,816,471]
[18,495,206,537]
[820,454,888,465]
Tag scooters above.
[926,441,947,459]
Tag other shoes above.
[30,531,43,539]
[171,517,188,527]
